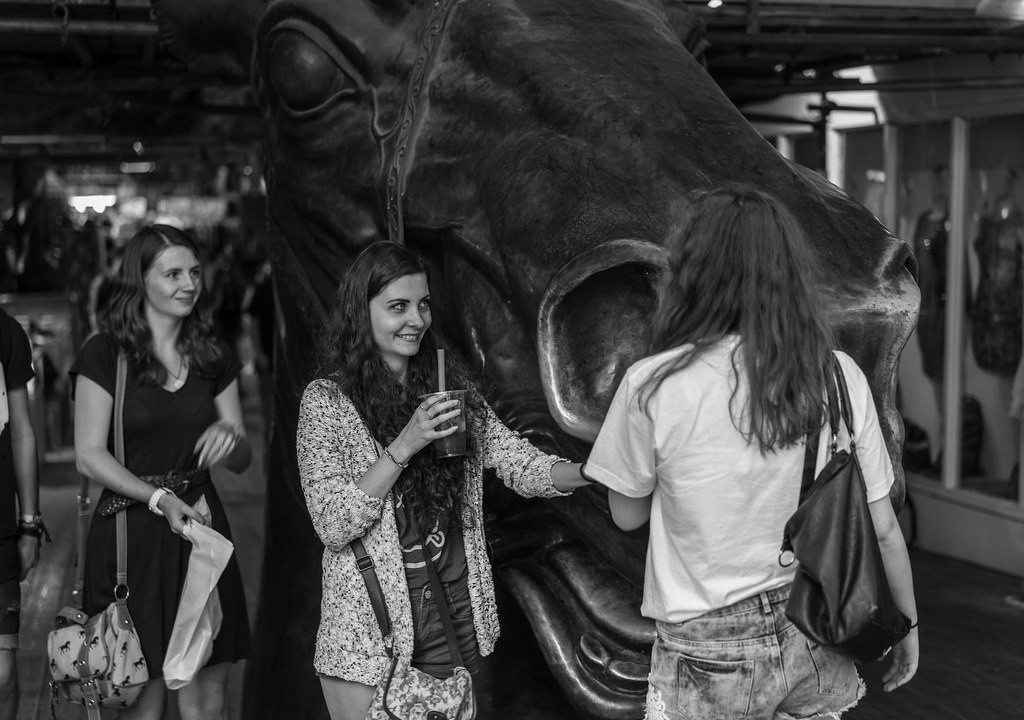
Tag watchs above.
[148,487,177,517]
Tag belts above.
[99,469,211,516]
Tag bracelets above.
[580,463,598,483]
[384,447,409,469]
[911,621,919,629]
[18,511,52,543]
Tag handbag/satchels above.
[779,350,911,663]
[364,656,478,720]
[47,598,150,719]
[164,494,234,691]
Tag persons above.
[583,182,921,720]
[0,305,52,720]
[67,224,255,720]
[294,239,599,720]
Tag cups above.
[419,388,471,460]
